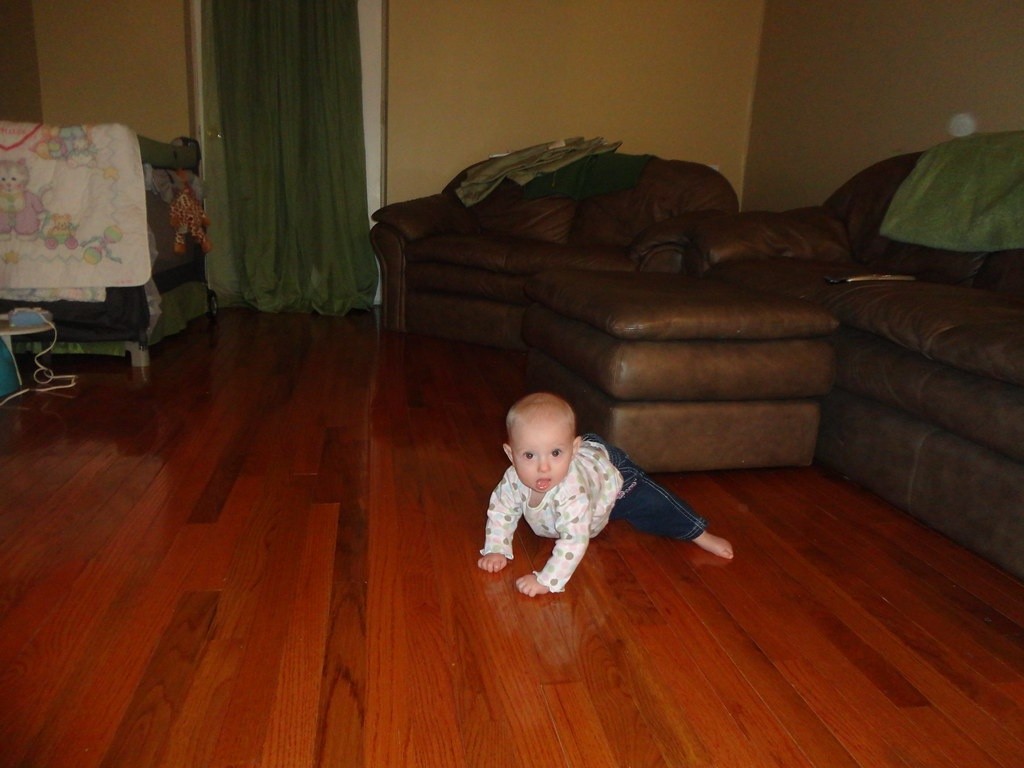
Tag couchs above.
[681,131,1024,586]
[368,152,740,352]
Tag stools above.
[521,270,839,474]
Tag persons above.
[477,393,733,598]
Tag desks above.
[0,320,54,385]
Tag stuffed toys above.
[155,168,211,253]
